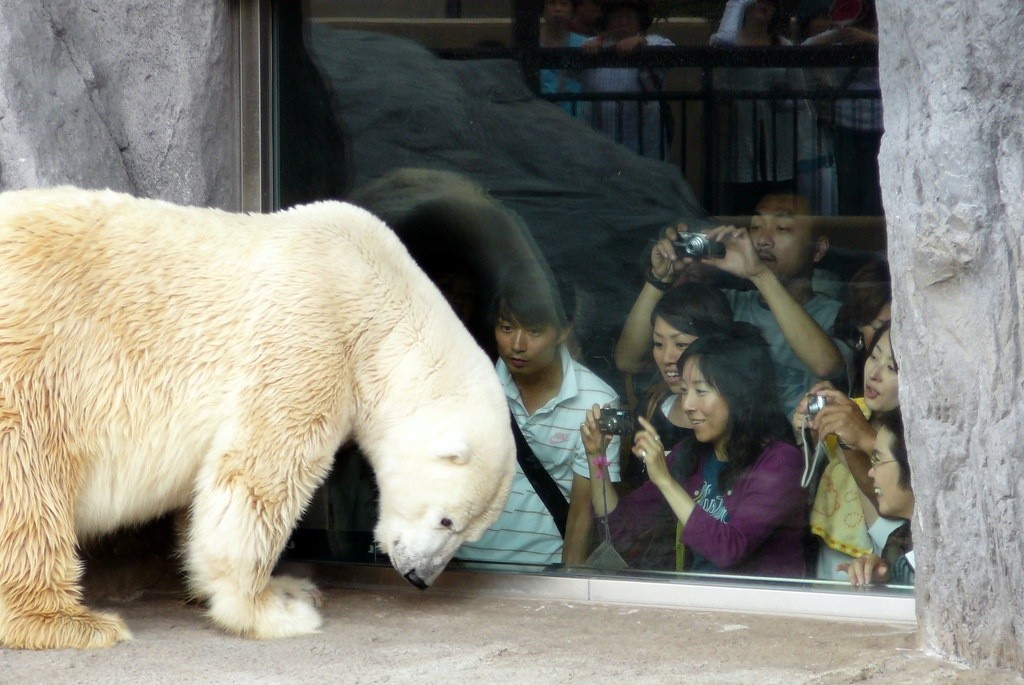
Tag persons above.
[611,177,871,588]
[580,261,915,592]
[443,258,623,575]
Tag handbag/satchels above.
[584,540,633,578]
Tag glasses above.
[869,455,898,470]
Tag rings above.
[642,450,647,458]
[654,434,661,440]
[580,423,587,428]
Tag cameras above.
[596,409,636,435]
[805,391,832,420]
[669,231,719,260]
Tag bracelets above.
[646,271,672,291]
